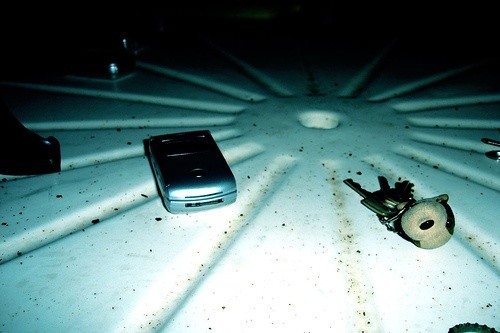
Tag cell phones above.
[143,128,238,215]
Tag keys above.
[341,173,455,250]
[480,136,500,147]
